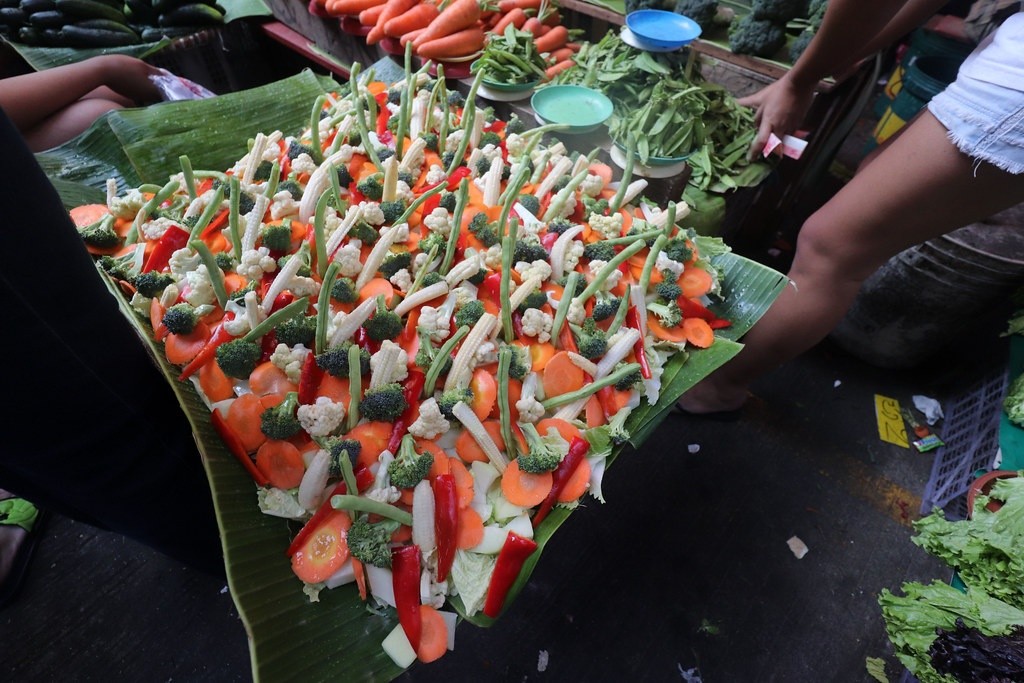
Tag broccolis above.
[625,0,846,67]
[76,63,729,569]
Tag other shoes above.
[668,398,742,422]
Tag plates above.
[606,143,688,179]
[533,113,605,135]
[608,118,700,166]
[531,84,614,131]
[624,9,703,48]
[619,26,682,52]
[475,61,546,92]
[473,84,533,101]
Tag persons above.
[665,0,1024,416]
[0,54,226,580]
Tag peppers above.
[532,438,589,527]
[139,89,731,659]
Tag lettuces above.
[879,373,1024,683]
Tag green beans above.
[557,29,777,198]
[471,24,548,87]
[496,349,519,462]
[500,236,516,342]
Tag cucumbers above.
[0,0,226,45]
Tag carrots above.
[230,396,267,451]
[542,355,584,401]
[258,440,305,488]
[326,0,589,78]
